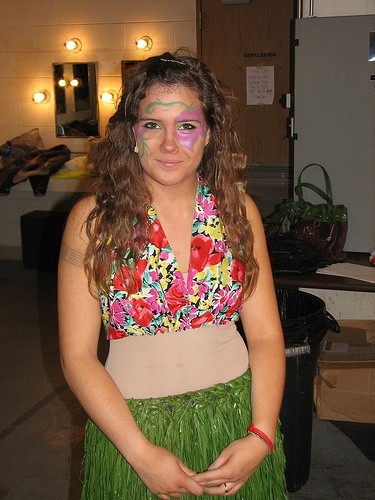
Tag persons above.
[57,52,289,500]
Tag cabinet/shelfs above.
[195,0,296,217]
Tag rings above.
[224,483,229,493]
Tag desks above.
[274,251,375,293]
[11,173,103,192]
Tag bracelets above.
[247,423,274,456]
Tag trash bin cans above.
[233,287,327,490]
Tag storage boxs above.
[311,318,375,423]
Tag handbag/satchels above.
[262,164,348,263]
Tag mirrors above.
[121,59,142,84]
[51,62,102,138]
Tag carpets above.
[329,420,375,462]
